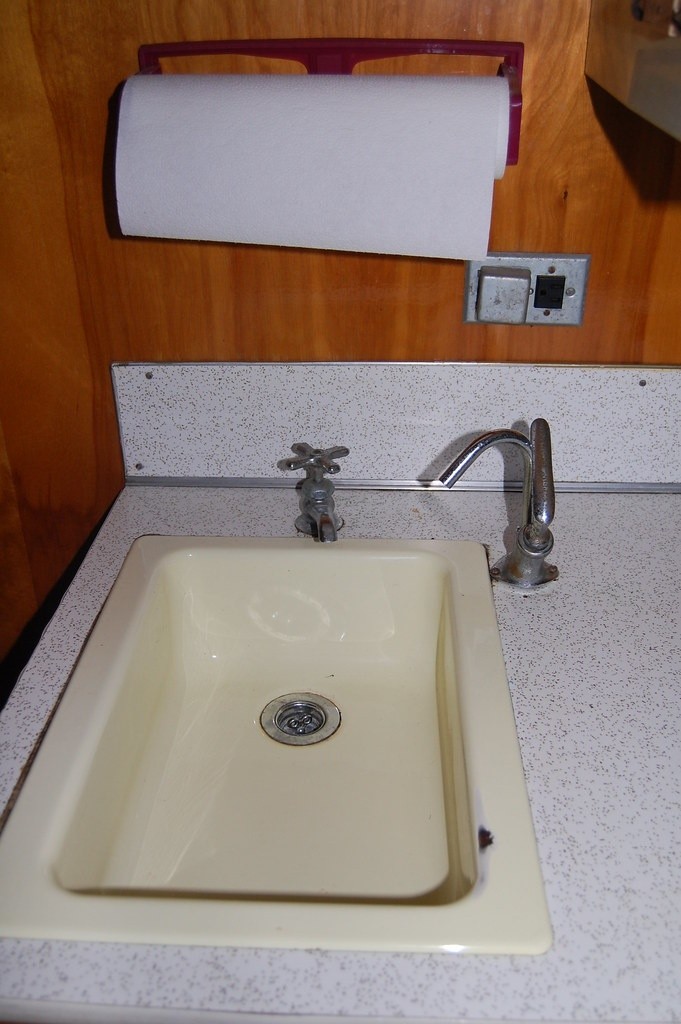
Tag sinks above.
[1,530,559,955]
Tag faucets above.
[440,416,562,589]
[283,436,352,546]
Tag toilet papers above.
[114,68,509,265]
